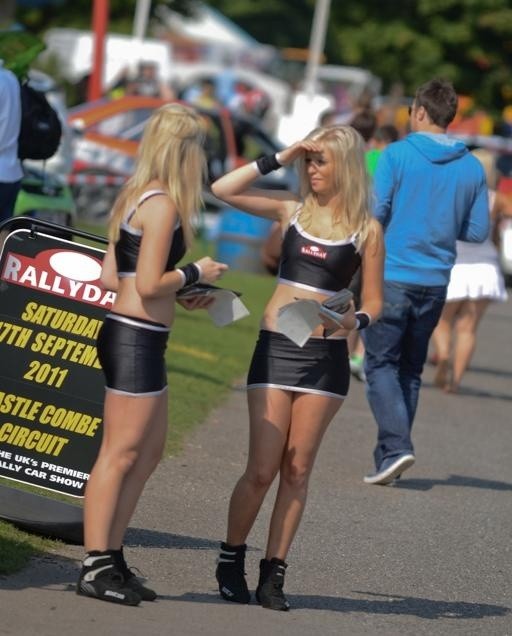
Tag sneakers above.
[363,452,415,484]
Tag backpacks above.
[17,73,61,159]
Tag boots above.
[216,542,250,603]
[78,545,156,605]
[255,558,290,611]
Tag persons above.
[354,78,411,172]
[76,101,228,606]
[430,148,508,390]
[359,78,491,487]
[125,61,171,98]
[210,125,385,612]
[0,49,25,247]
[192,79,221,108]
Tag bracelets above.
[256,152,283,175]
[175,263,201,288]
[355,311,372,330]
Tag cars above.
[15,93,290,221]
